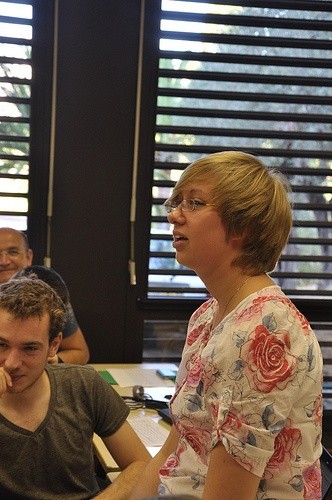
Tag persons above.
[0,227,90,364]
[125,151,324,500]
[0,279,153,500]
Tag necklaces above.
[212,274,254,332]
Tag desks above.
[90,363,179,483]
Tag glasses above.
[165,199,217,212]
[0,248,27,257]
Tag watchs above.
[57,354,64,363]
